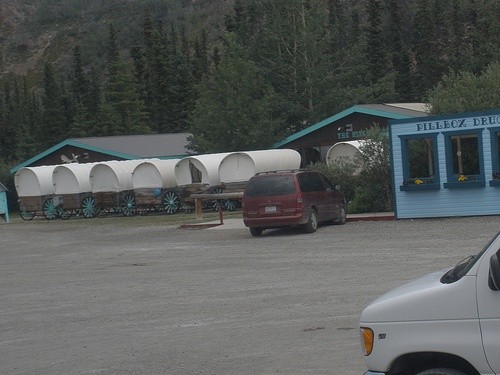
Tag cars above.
[358,227,500,375]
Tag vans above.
[242,169,346,238]
